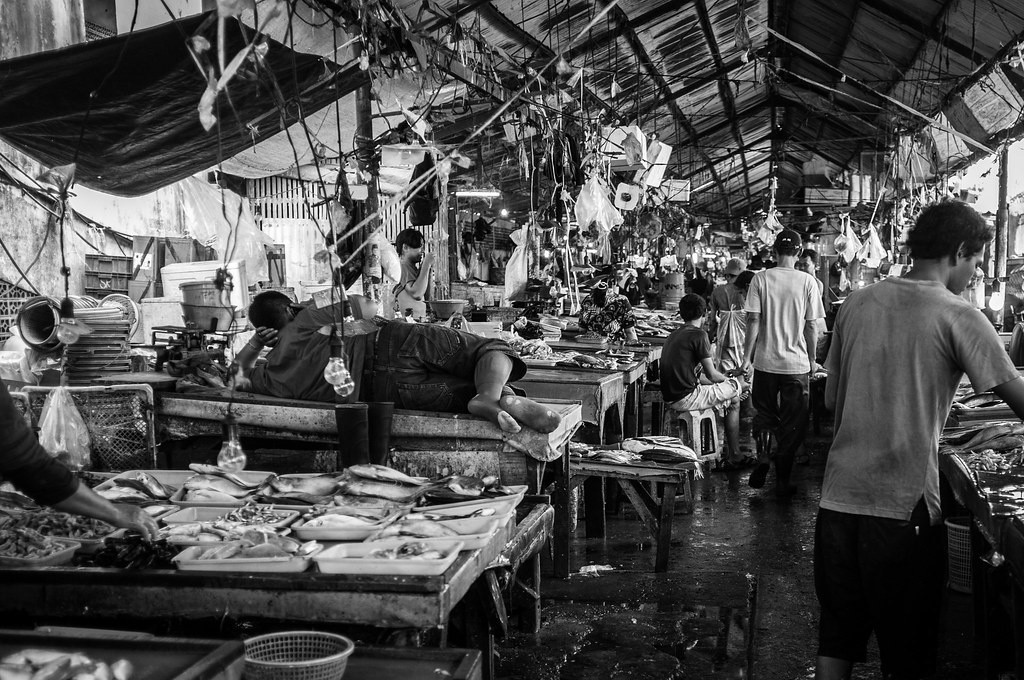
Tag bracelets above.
[248,339,264,350]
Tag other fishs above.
[0,648,134,680]
[97,462,517,561]
[940,422,1024,455]
[951,382,1003,409]
[639,448,702,463]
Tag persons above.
[391,228,438,318]
[578,266,638,341]
[0,378,160,543]
[741,229,826,498]
[225,290,562,434]
[813,201,1024,680]
[660,250,850,467]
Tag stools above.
[662,409,722,470]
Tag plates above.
[0,465,529,576]
[519,316,562,341]
[520,354,565,366]
[574,335,607,344]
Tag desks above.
[0,313,687,680]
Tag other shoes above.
[730,453,756,467]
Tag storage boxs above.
[631,137,672,187]
[598,125,648,172]
[83,234,249,344]
[805,187,850,205]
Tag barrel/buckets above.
[16,295,137,447]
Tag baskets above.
[944,517,974,580]
[241,631,355,680]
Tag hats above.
[724,258,747,276]
[774,229,802,253]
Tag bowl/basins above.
[429,300,467,318]
[179,282,236,332]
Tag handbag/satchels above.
[36,385,90,471]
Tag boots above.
[774,452,797,502]
[750,429,772,490]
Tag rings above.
[257,333,263,337]
[744,373,746,376]
